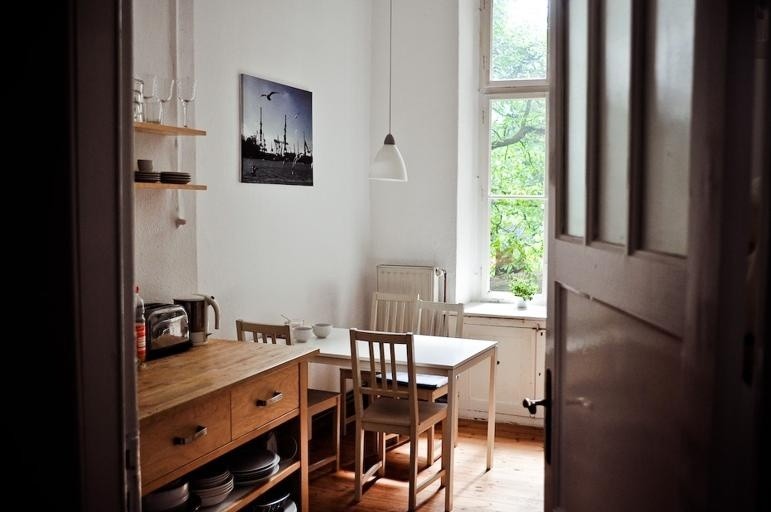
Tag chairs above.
[344,326,454,509]
[378,300,465,466]
[367,293,420,334]
[234,317,344,486]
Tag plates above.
[229,447,281,486]
[134,171,161,183]
[161,172,191,184]
[192,468,235,506]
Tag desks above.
[135,339,320,512]
[256,320,499,511]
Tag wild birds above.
[260,91,279,101]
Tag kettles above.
[173,293,220,345]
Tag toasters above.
[141,302,192,360]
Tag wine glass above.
[178,76,197,127]
[139,75,156,124]
[156,78,174,125]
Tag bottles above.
[135,286,145,365]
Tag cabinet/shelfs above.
[133,117,209,194]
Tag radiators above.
[376,264,438,335]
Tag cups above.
[137,160,154,171]
[284,319,303,330]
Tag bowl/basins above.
[293,326,313,343]
[268,433,297,464]
[313,322,333,339]
[146,482,190,508]
[252,489,292,511]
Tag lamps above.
[365,1,409,186]
